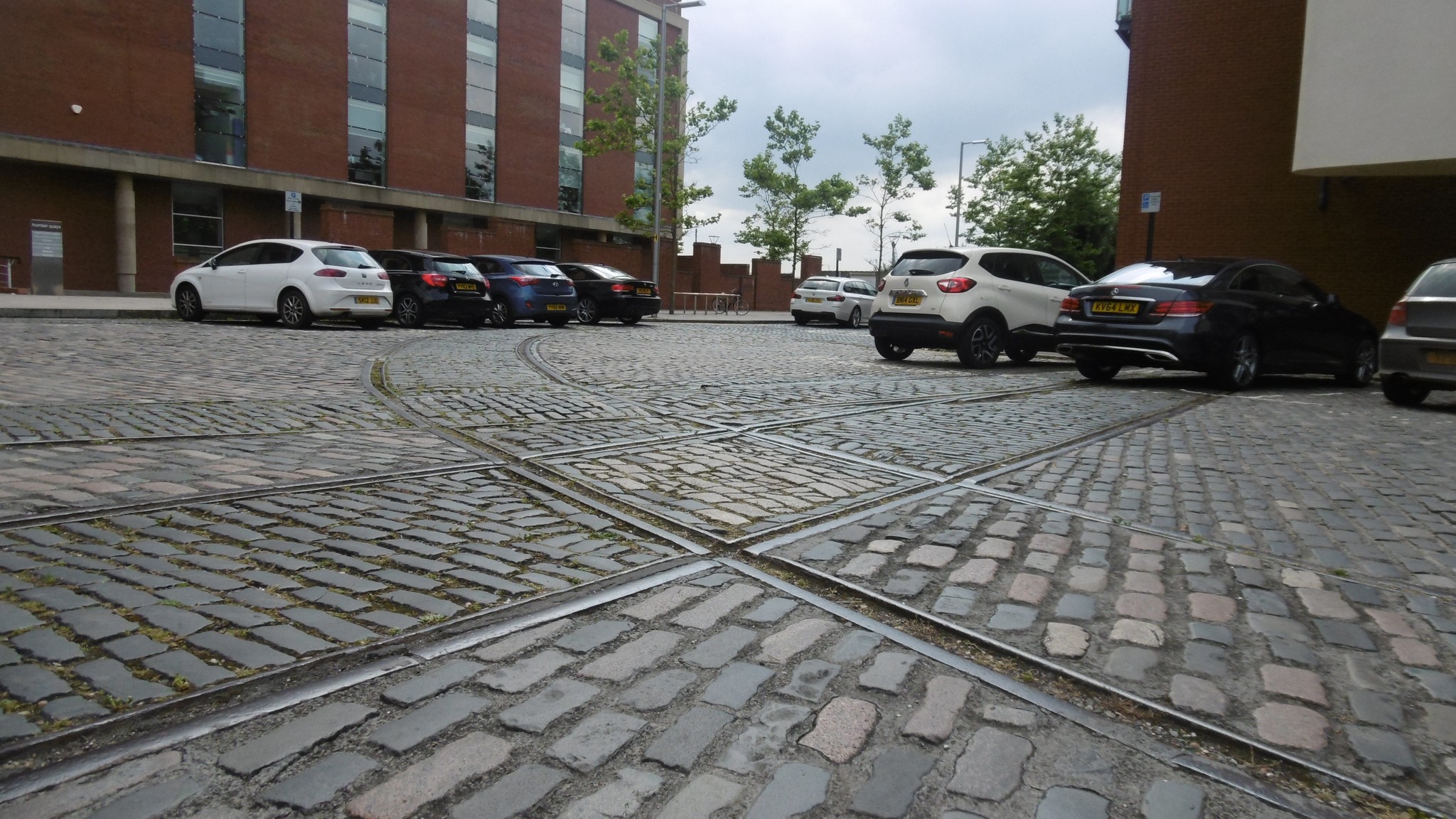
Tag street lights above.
[888,235,900,268]
[652,1,706,317]
[955,140,987,247]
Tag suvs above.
[868,248,1094,370]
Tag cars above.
[366,249,491,329]
[466,255,578,329]
[790,275,878,329]
[1377,258,1456,407]
[1052,257,1379,393]
[555,263,661,326]
[170,239,393,330]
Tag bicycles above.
[712,288,750,315]
